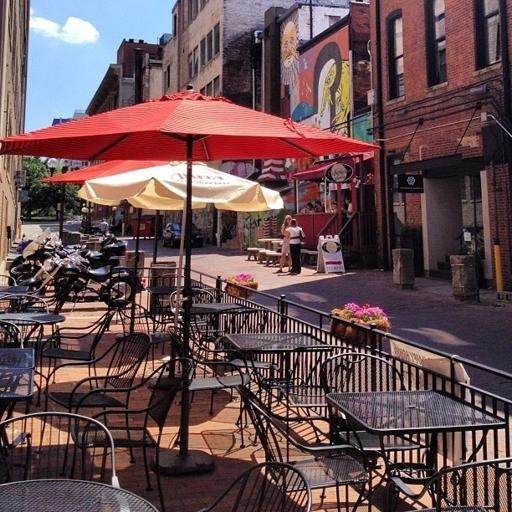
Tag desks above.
[257,237,305,251]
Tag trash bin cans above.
[392,249,415,290]
[148,262,176,314]
[125,251,144,277]
[450,254,480,304]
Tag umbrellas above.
[0,83,386,476]
[75,158,286,394]
[41,155,216,345]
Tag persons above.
[342,195,352,248]
[284,218,306,275]
[273,214,293,274]
[99,218,110,240]
[297,196,337,215]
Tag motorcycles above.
[8,231,138,310]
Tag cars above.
[161,221,206,247]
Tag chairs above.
[0,276,511,512]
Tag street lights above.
[82,206,93,234]
[44,156,68,242]
[111,207,116,228]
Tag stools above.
[247,248,318,267]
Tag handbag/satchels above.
[299,230,304,242]
